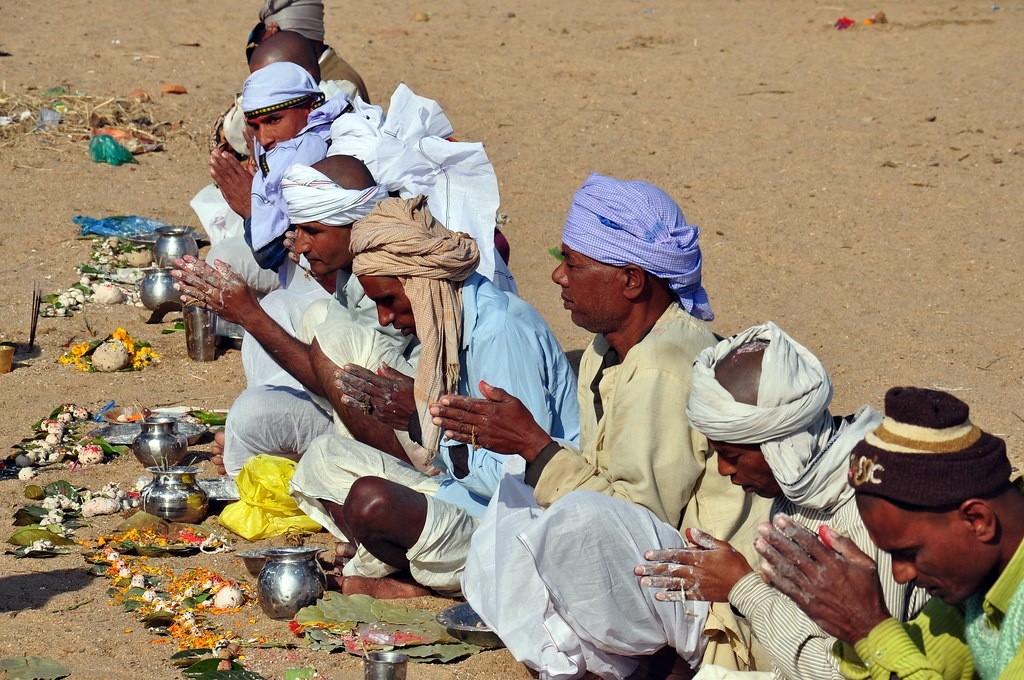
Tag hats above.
[848,383,1010,511]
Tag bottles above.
[257,547,328,618]
[133,417,188,469]
[141,268,182,311]
[152,225,198,268]
[140,466,209,525]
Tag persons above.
[172,0,579,601]
[430,174,775,680]
[634,320,932,680]
[753,387,1024,680]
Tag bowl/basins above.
[436,601,505,647]
[234,548,267,579]
[196,478,241,510]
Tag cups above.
[183,303,215,362]
[361,651,409,680]
[0,345,16,373]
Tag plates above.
[87,422,208,448]
